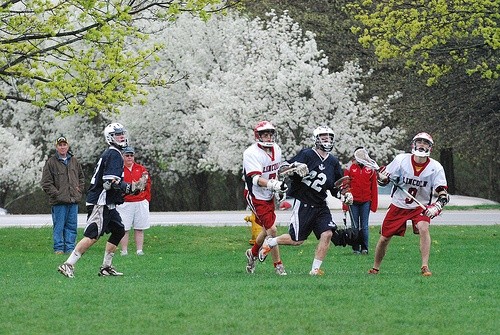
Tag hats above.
[56,137,67,146]
[122,147,135,155]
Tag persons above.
[116,148,151,256]
[258,125,353,275]
[40,136,86,254]
[241,121,288,275]
[58,123,149,278]
[368,132,450,276]
[341,147,379,255]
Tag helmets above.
[411,133,433,157]
[105,123,129,151]
[313,126,334,153]
[254,121,275,147]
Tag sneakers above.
[58,262,75,277]
[98,266,123,276]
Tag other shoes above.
[275,265,287,275]
[54,250,65,255]
[258,236,273,263]
[246,249,256,274]
[135,249,145,255]
[310,268,322,275]
[120,250,128,256]
[420,266,431,276]
[369,268,379,274]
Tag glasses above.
[126,155,134,157]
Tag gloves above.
[341,192,353,206]
[420,202,443,219]
[267,180,288,192]
[377,166,390,186]
[121,181,140,196]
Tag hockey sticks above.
[123,172,148,199]
[355,149,428,211]
[334,175,361,228]
[278,164,295,203]
